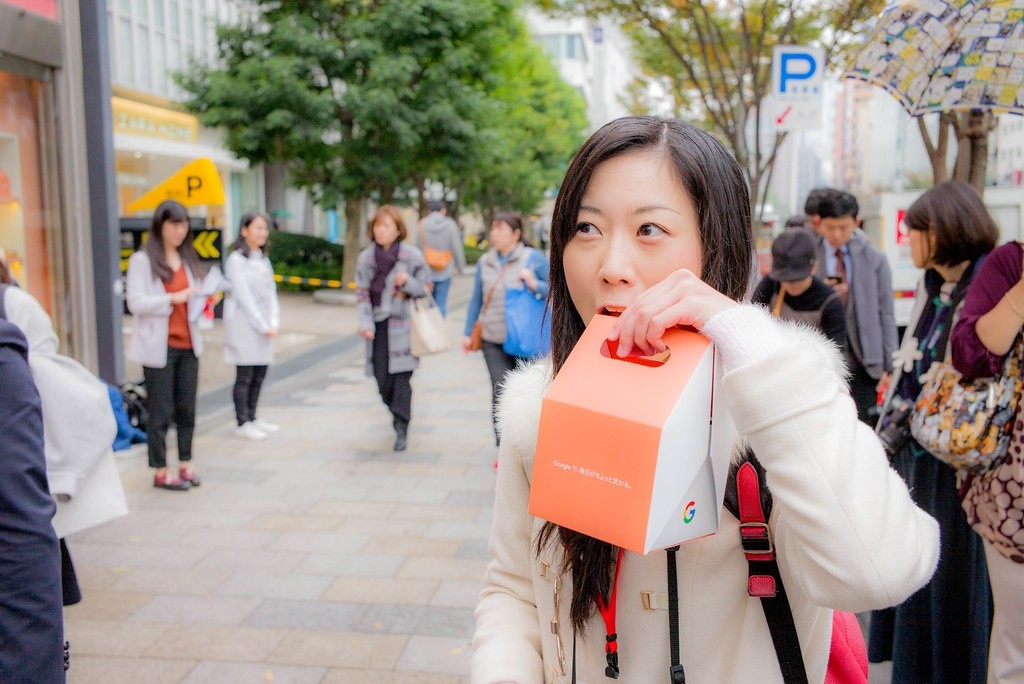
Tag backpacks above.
[728,446,869,683]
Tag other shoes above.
[395,432,408,451]
[236,422,267,441]
[155,476,192,490]
[250,417,275,433]
[178,469,199,487]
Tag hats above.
[770,230,816,281]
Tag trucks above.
[862,187,1024,328]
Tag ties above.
[834,249,848,304]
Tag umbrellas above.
[839,0,1024,115]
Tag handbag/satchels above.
[425,248,452,271]
[469,324,484,352]
[505,260,555,359]
[407,282,454,358]
[907,362,1019,474]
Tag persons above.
[469,117,940,684]
[224,214,279,438]
[124,201,219,490]
[747,181,1024,684]
[0,266,119,684]
[535,209,549,251]
[463,213,557,471]
[354,202,436,452]
[415,200,466,318]
